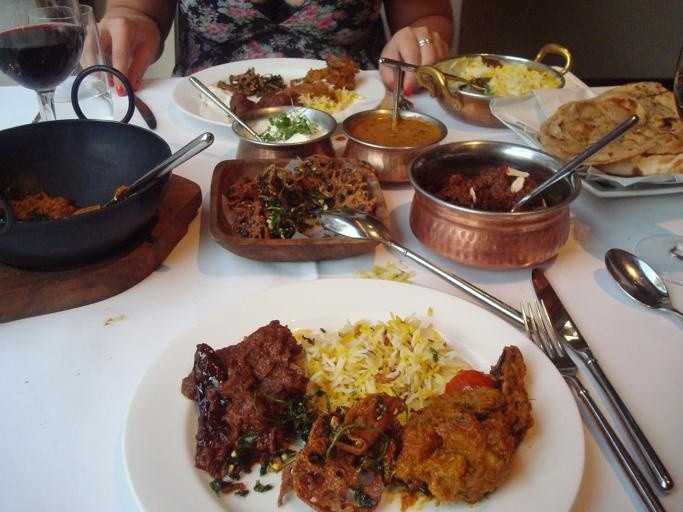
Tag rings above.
[417,37,434,48]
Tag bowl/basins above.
[232,106,337,162]
[407,140,585,272]
[341,106,447,185]
[209,158,390,263]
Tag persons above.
[79,0,473,97]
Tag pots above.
[0,64,180,273]
[415,43,574,128]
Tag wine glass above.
[636,42,683,285]
[0,0,87,119]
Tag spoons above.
[604,247,683,318]
[317,208,532,325]
[377,56,497,89]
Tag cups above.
[28,4,114,122]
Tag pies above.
[539,81,683,177]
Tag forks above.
[398,95,413,110]
[519,299,667,512]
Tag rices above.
[431,56,561,98]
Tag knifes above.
[133,95,157,129]
[532,268,674,490]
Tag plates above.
[170,56,386,128]
[578,173,683,199]
[121,279,585,511]
